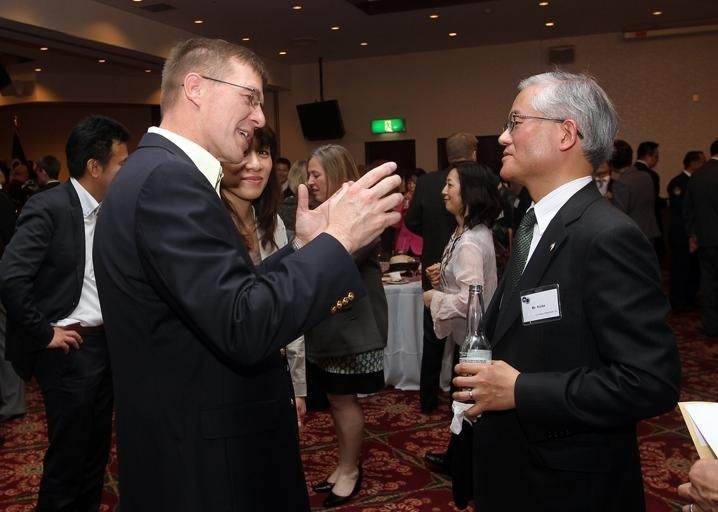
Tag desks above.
[381,264,455,391]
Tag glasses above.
[504,111,584,140]
[182,75,260,109]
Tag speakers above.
[295,99,346,141]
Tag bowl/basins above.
[389,261,419,277]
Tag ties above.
[502,208,537,302]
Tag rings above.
[469,391,472,400]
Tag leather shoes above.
[427,454,464,470]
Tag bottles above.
[459,285,492,404]
[20,177,37,193]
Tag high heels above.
[314,467,362,506]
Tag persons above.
[354,160,427,258]
[453,70,682,512]
[405,133,502,414]
[93,36,404,511]
[219,124,306,433]
[306,143,389,508]
[490,181,533,252]
[423,160,497,474]
[594,139,718,342]
[1,118,133,511]
[276,157,308,237]
